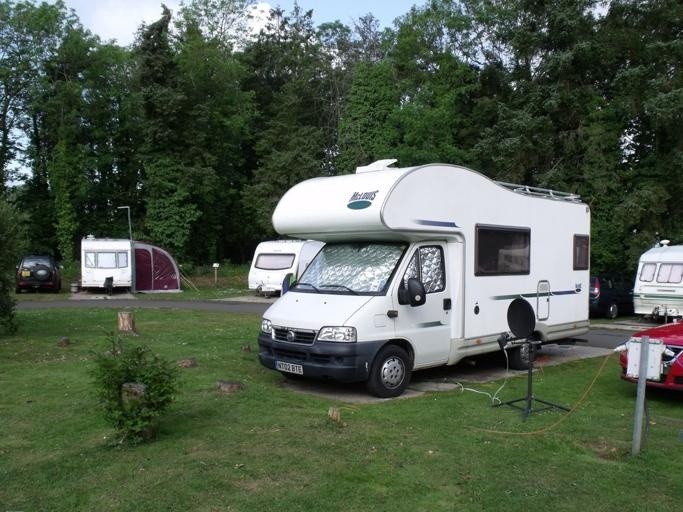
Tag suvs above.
[15,256,61,294]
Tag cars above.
[619,319,683,392]
[590,272,634,319]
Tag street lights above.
[117,206,139,294]
